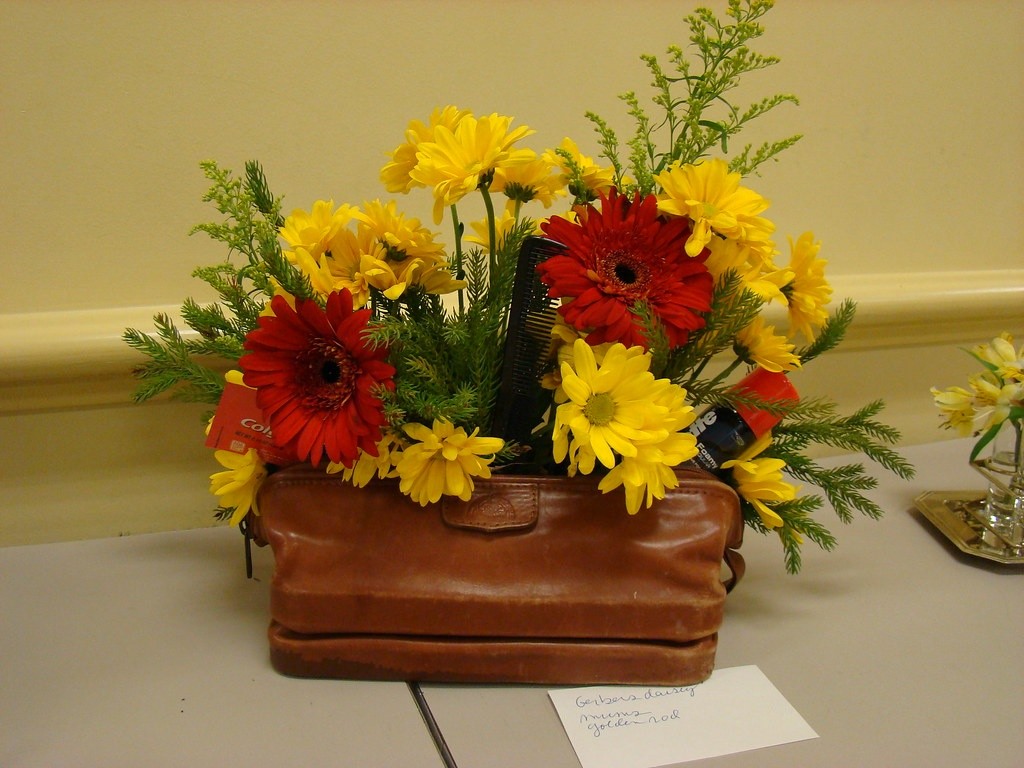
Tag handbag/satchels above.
[237,464,745,686]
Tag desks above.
[1,437,1023,768]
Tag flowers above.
[119,1,918,571]
[930,327,1024,458]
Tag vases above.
[981,424,1024,552]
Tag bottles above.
[677,365,802,478]
